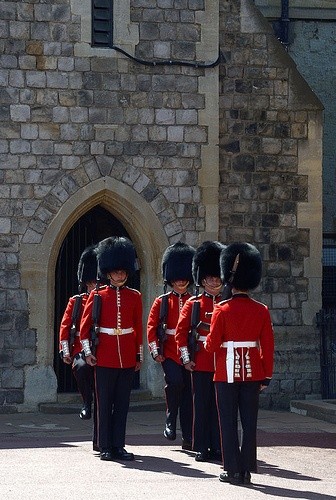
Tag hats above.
[97,237,136,277]
[192,240,227,284]
[162,241,196,288]
[220,242,262,291]
[77,245,98,283]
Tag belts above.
[222,341,256,383]
[76,332,80,336]
[198,336,207,341]
[165,329,175,335]
[99,327,134,335]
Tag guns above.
[90,260,102,364]
[197,253,241,331]
[69,263,85,354]
[157,264,168,355]
[188,266,200,369]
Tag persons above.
[147,280,195,450]
[175,269,223,462]
[80,270,144,461]
[59,278,105,419]
[203,286,275,486]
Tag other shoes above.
[182,443,200,452]
[106,451,135,461]
[80,407,92,420]
[219,469,251,484]
[164,423,176,440]
[100,448,127,460]
[195,450,222,462]
